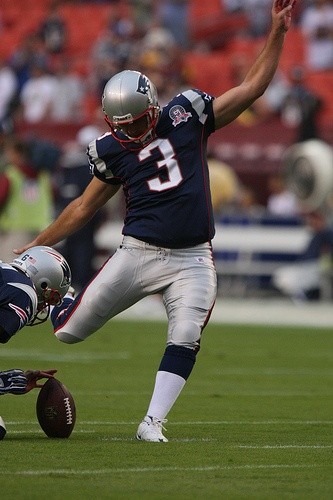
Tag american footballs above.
[36,378,77,441]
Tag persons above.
[0,0,333,304]
[13,0,300,445]
[0,245,71,441]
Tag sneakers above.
[63,286,75,298]
[135,416,169,443]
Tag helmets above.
[9,245,71,306]
[102,71,159,126]
